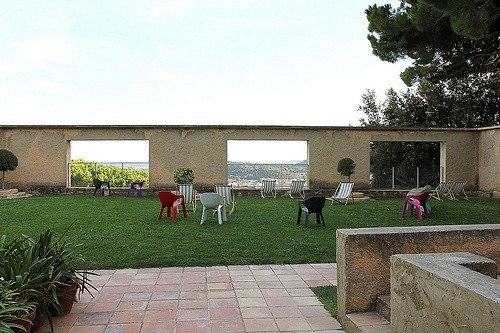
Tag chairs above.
[157,191,187,222]
[297,197,326,227]
[287,180,306,200]
[402,181,469,221]
[93,179,111,196]
[175,182,197,214]
[212,184,237,215]
[200,193,227,225]
[128,180,144,199]
[326,182,355,206]
[260,180,276,199]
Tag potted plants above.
[0,219,102,333]
